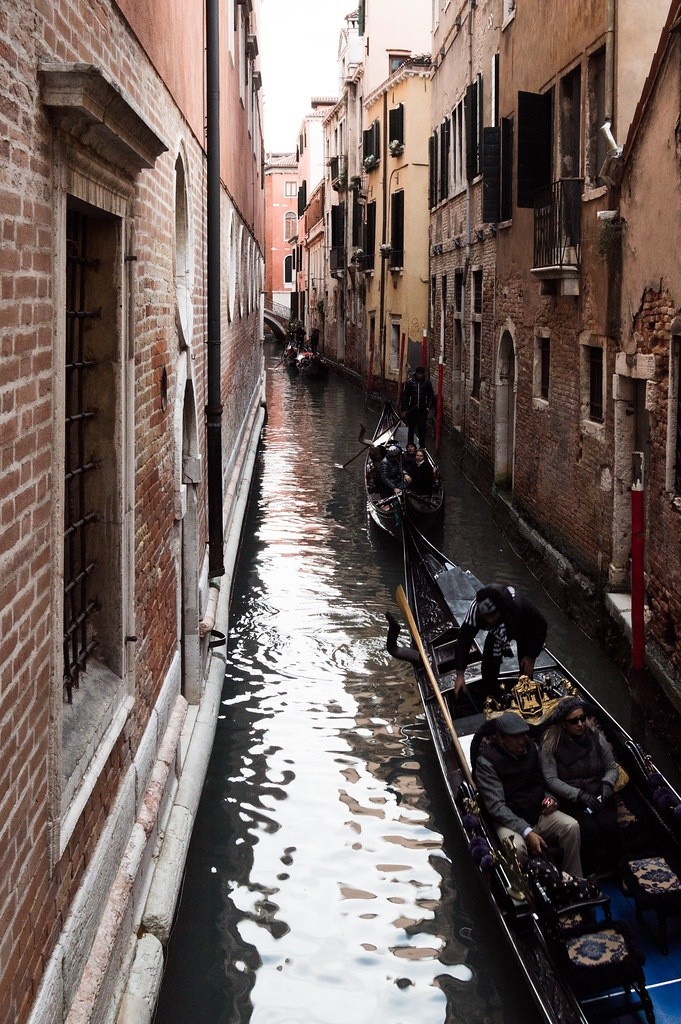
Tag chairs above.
[527,863,614,930]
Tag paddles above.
[394,584,478,795]
[275,339,311,368]
[343,404,419,468]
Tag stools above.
[621,851,681,956]
[558,918,656,1024]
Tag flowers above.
[388,140,405,154]
[364,154,375,166]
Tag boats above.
[282,342,304,368]
[391,507,680,1024]
[295,351,329,381]
[365,405,443,551]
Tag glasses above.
[566,715,585,723]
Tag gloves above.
[601,783,614,802]
[577,791,603,811]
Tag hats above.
[495,711,529,735]
[475,584,504,611]
[549,696,585,720]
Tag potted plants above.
[357,249,364,258]
[381,244,394,259]
[350,175,360,183]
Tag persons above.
[284,342,321,370]
[472,695,623,884]
[368,441,435,497]
[451,586,549,694]
